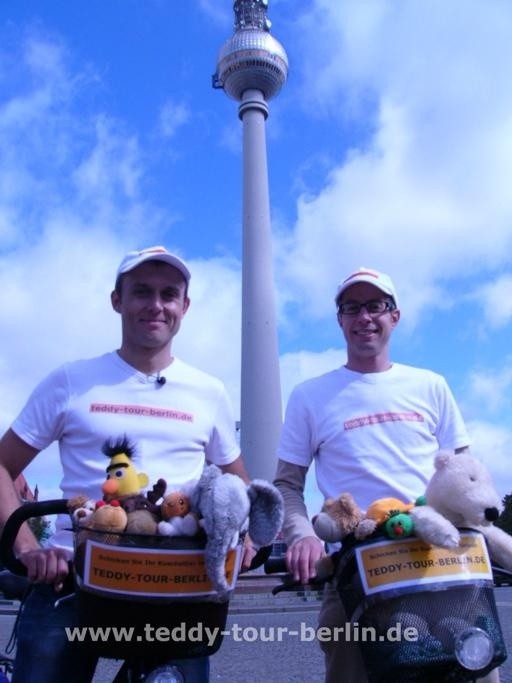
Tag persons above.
[67,437,285,604]
[0,246,254,683]
[272,267,499,683]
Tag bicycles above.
[0,495,276,683]
[263,535,512,681]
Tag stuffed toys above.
[311,449,512,654]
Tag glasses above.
[338,301,394,313]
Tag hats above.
[336,267,398,308]
[116,246,190,297]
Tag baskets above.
[331,528,507,678]
[74,524,249,657]
